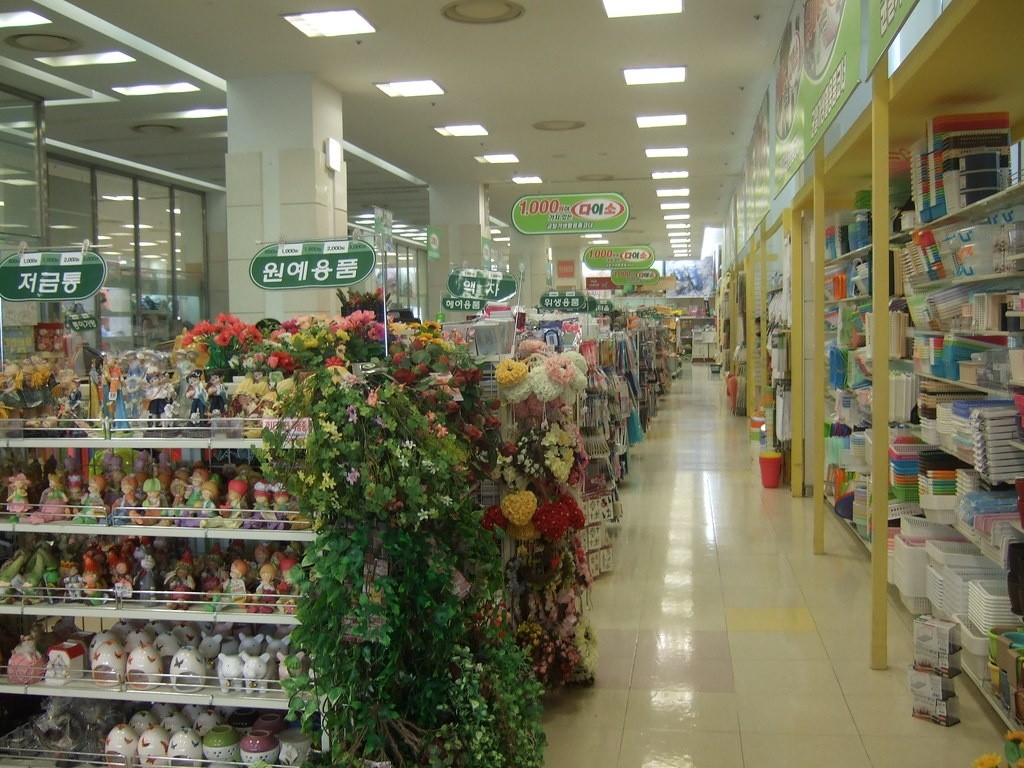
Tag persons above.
[0,351,325,614]
[612,310,627,332]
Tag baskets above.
[848,428,1024,680]
[710,364,721,374]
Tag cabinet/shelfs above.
[0,416,338,767]
[675,314,716,354]
[824,177,1024,742]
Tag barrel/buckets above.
[759,453,782,488]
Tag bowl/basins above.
[987,627,1024,722]
[203,706,286,753]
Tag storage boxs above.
[710,364,721,373]
[824,111,1022,729]
